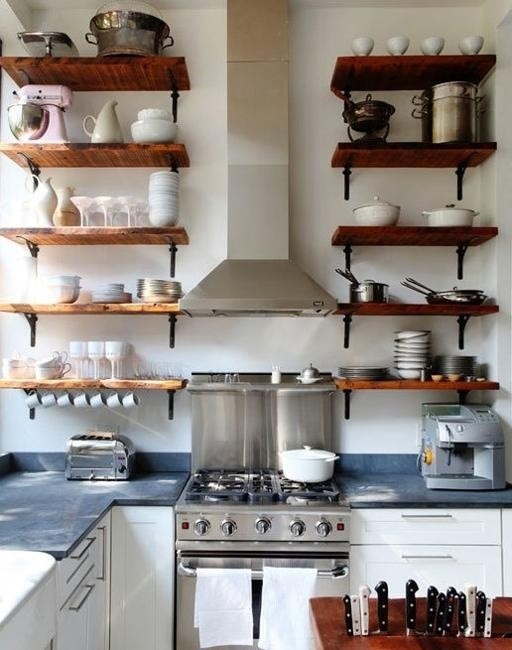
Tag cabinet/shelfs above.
[0,507,175,650]
[330,54,501,419]
[347,507,512,598]
[0,55,193,421]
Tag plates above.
[296,376,322,385]
[432,355,475,380]
[87,283,133,305]
[338,365,391,381]
[136,277,182,303]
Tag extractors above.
[179,1,340,320]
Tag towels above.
[258,566,317,650]
[193,567,254,649]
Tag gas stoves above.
[173,469,351,506]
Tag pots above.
[277,445,339,482]
[336,267,388,304]
[421,203,478,229]
[84,1,174,56]
[400,274,486,304]
[353,195,400,227]
[411,80,485,144]
[342,86,395,142]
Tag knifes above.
[442,587,458,638]
[359,583,371,636]
[476,591,486,637]
[371,581,389,636]
[483,593,493,638]
[406,579,419,636]
[425,585,439,634]
[434,593,445,636]
[457,591,466,637]
[465,584,477,638]
[351,595,361,637]
[343,594,353,635]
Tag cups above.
[73,389,91,408]
[26,390,41,408]
[131,360,191,380]
[122,390,140,408]
[40,390,57,406]
[2,346,71,383]
[89,390,106,408]
[57,388,74,407]
[106,390,124,408]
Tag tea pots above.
[83,99,125,145]
[22,172,59,229]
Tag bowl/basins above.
[420,36,445,56]
[386,36,409,55]
[393,326,430,381]
[148,171,180,226]
[44,275,81,305]
[460,35,485,55]
[447,374,461,381]
[131,120,180,145]
[349,37,373,56]
[432,375,443,382]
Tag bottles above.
[271,365,282,385]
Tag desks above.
[310,596,512,650]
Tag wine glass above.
[70,194,147,227]
[69,339,129,379]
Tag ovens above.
[173,511,349,650]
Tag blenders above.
[6,84,73,144]
